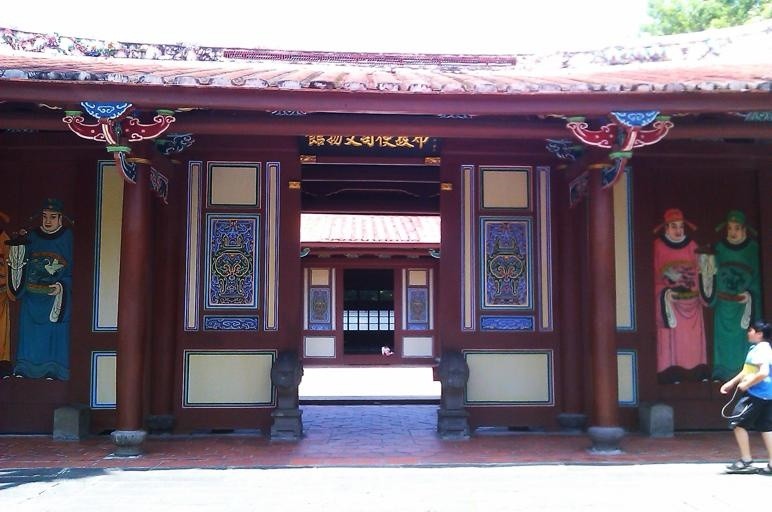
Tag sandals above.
[727,460,772,474]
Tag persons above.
[720,319,772,475]
[0,196,74,381]
[381,344,395,356]
[649,208,760,385]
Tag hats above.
[714,210,759,237]
[652,207,698,236]
[29,198,76,224]
